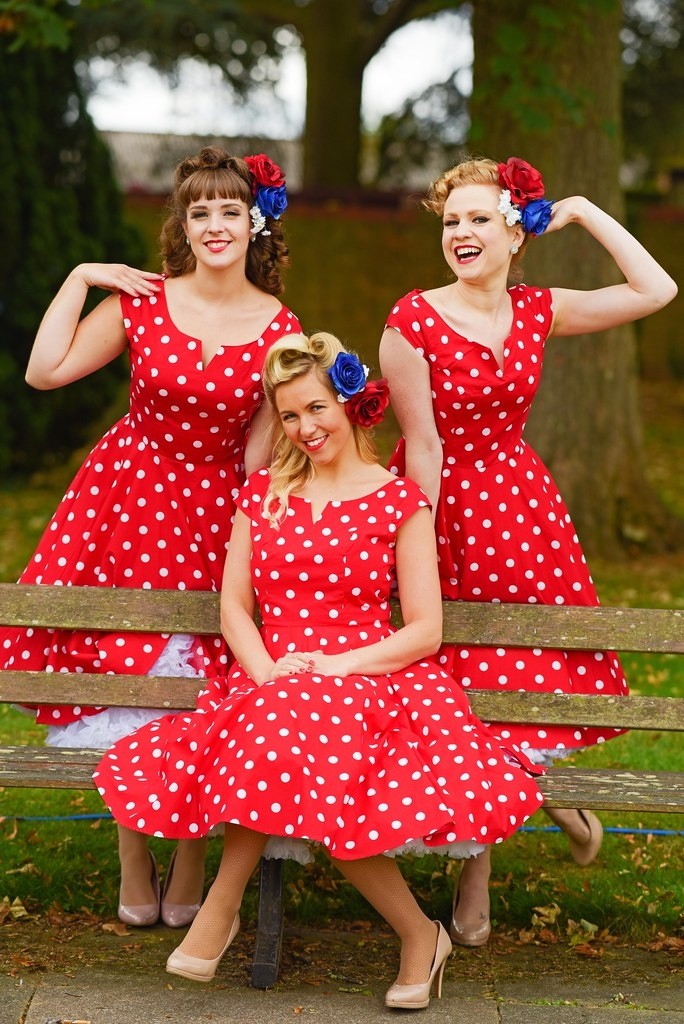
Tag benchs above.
[0,583,684,993]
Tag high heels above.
[383,919,453,1008]
[447,878,492,948]
[165,911,241,983]
[117,846,161,928]
[568,808,604,867]
[160,846,205,928]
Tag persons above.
[93,333,543,1009]
[377,158,678,947]
[0,147,302,927]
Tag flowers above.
[248,155,288,236]
[327,352,391,430]
[496,158,554,238]
